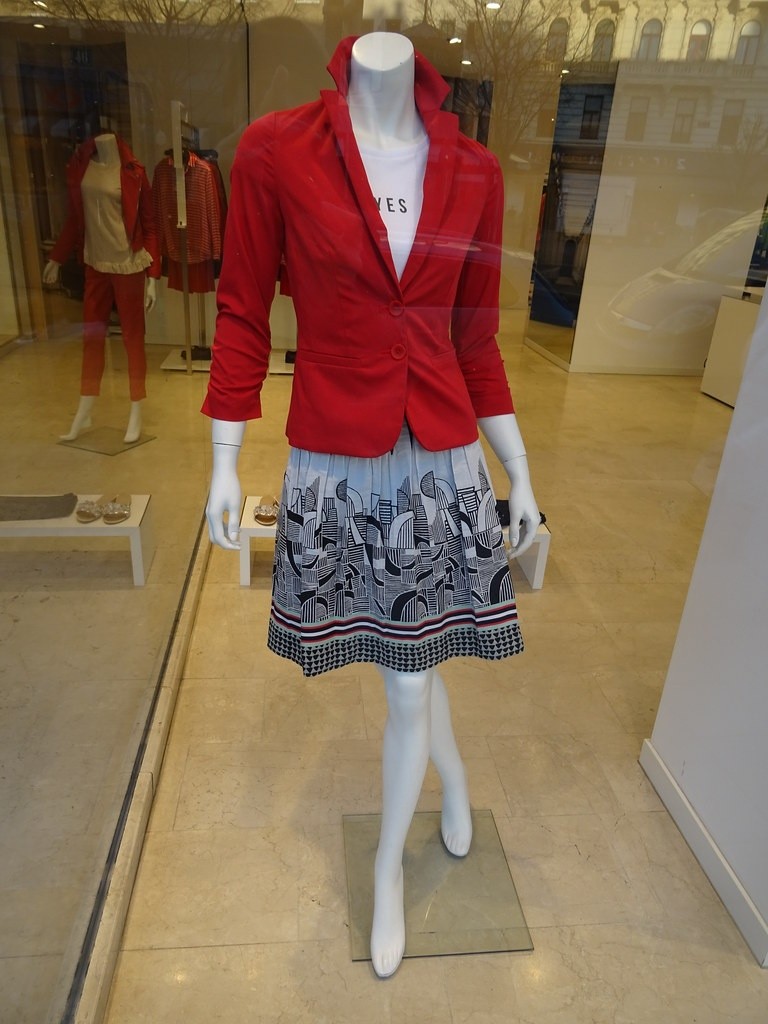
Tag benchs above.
[1,494,156,587]
[239,496,551,590]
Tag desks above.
[701,286,764,407]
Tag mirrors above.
[522,54,621,368]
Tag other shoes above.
[180,345,211,361]
[286,346,295,364]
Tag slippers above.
[253,495,283,526]
[76,489,134,525]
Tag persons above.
[42,133,162,444]
[199,30,542,979]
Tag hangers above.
[165,136,219,160]
